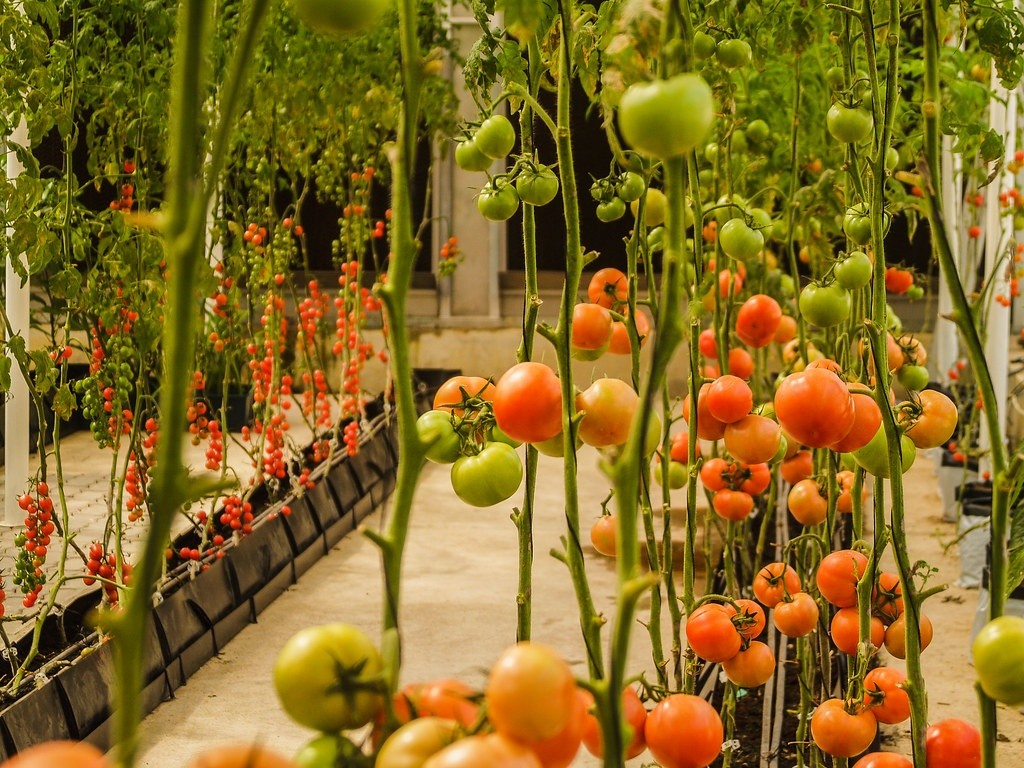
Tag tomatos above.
[0,27,1024,768]
